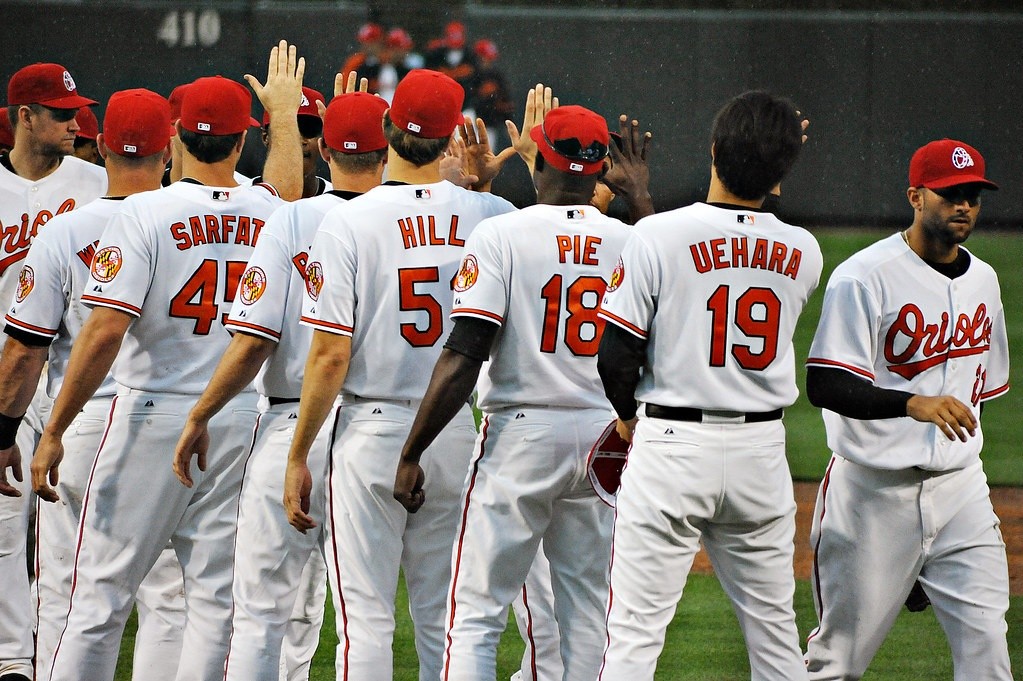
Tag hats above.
[263,85,325,126]
[355,21,500,62]
[102,89,178,158]
[168,84,188,123]
[387,68,466,137]
[75,107,100,140]
[8,61,101,110]
[0,108,15,146]
[322,90,392,152]
[529,104,610,176]
[180,75,261,135]
[908,136,1001,192]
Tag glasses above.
[51,109,78,121]
[541,119,609,165]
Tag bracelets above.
[0,411,27,450]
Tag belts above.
[646,403,785,423]
[268,396,301,406]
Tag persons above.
[283,67,563,681]
[392,102,656,681]
[0,40,562,681]
[803,139,1015,681]
[595,87,823,681]
[337,21,509,183]
